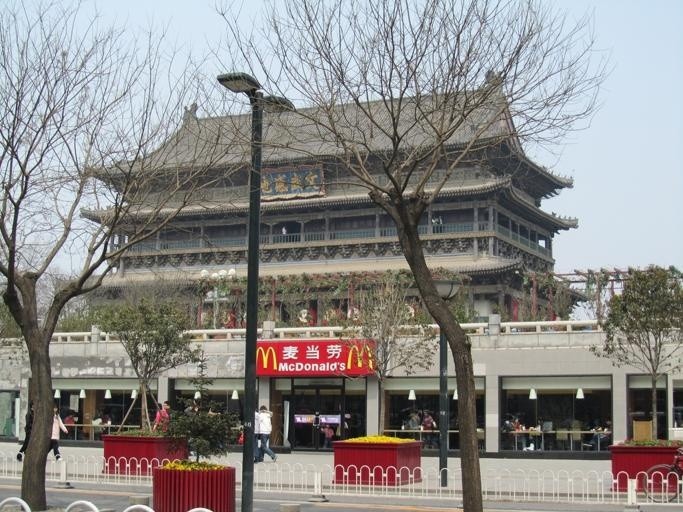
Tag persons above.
[321,424,335,448]
[388,410,612,453]
[16,399,280,463]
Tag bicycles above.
[642,446,682,503]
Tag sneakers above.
[56,455,62,462]
[17,452,22,459]
[253,456,279,464]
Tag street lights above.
[435,279,464,486]
[216,74,293,511]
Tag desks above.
[383,428,484,450]
[501,428,611,452]
[62,423,140,441]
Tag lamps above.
[407,388,460,401]
[528,386,586,400]
[52,389,138,400]
[193,389,239,400]
